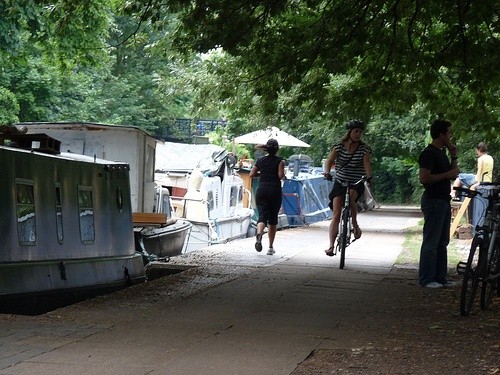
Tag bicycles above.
[452,172,500,317]
[323,175,372,270]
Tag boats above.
[183,187,255,255]
[132,182,193,266]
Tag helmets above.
[346,119,365,129]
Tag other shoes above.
[441,281,456,286]
[425,281,443,288]
[255,234,262,252]
[267,247,276,254]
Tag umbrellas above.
[233,125,311,151]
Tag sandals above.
[325,246,334,256]
[352,225,361,239]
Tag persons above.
[249,139,286,255]
[451,141,494,200]
[321,121,372,257]
[417,120,459,288]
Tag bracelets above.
[451,156,458,159]
